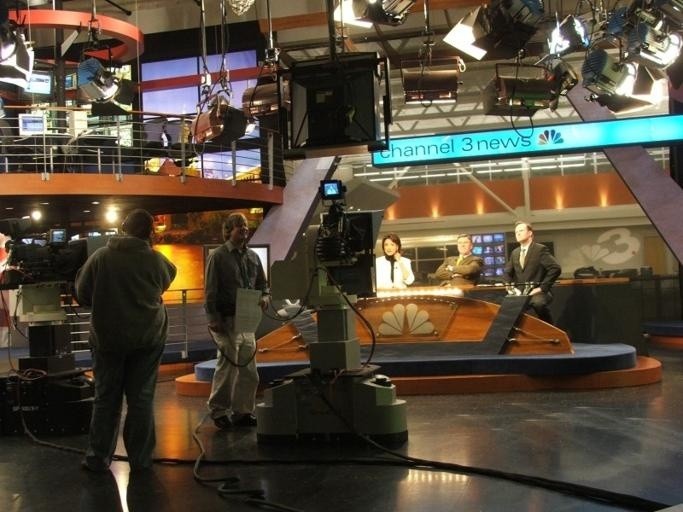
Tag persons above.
[503,221,562,323]
[435,234,484,286]
[74,208,177,473]
[204,212,272,429]
[376,233,415,288]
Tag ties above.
[456,256,463,266]
[520,248,525,269]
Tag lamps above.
[65,3,142,118]
[0,0,61,101]
[181,0,683,154]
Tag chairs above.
[136,115,199,166]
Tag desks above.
[13,135,120,174]
[629,273,679,320]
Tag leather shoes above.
[212,415,234,430]
[234,414,256,427]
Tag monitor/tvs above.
[63,72,77,92]
[203,242,272,287]
[21,70,56,97]
